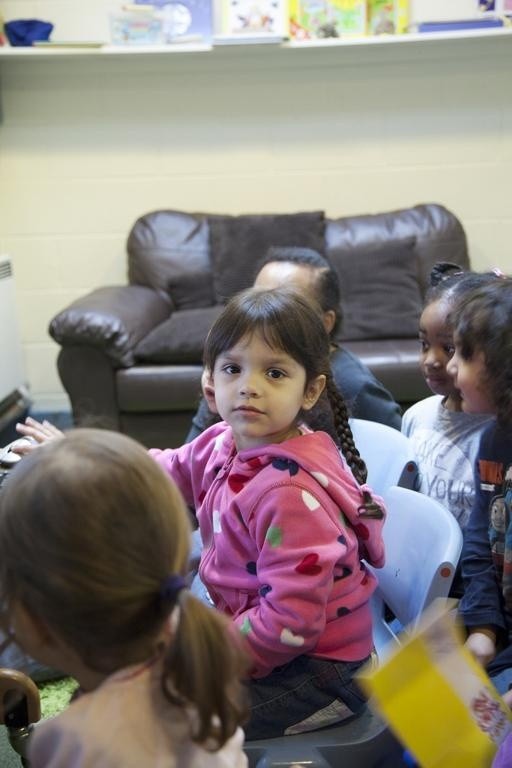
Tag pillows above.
[134,208,328,369]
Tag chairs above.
[190,416,464,768]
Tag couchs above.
[48,200,474,446]
